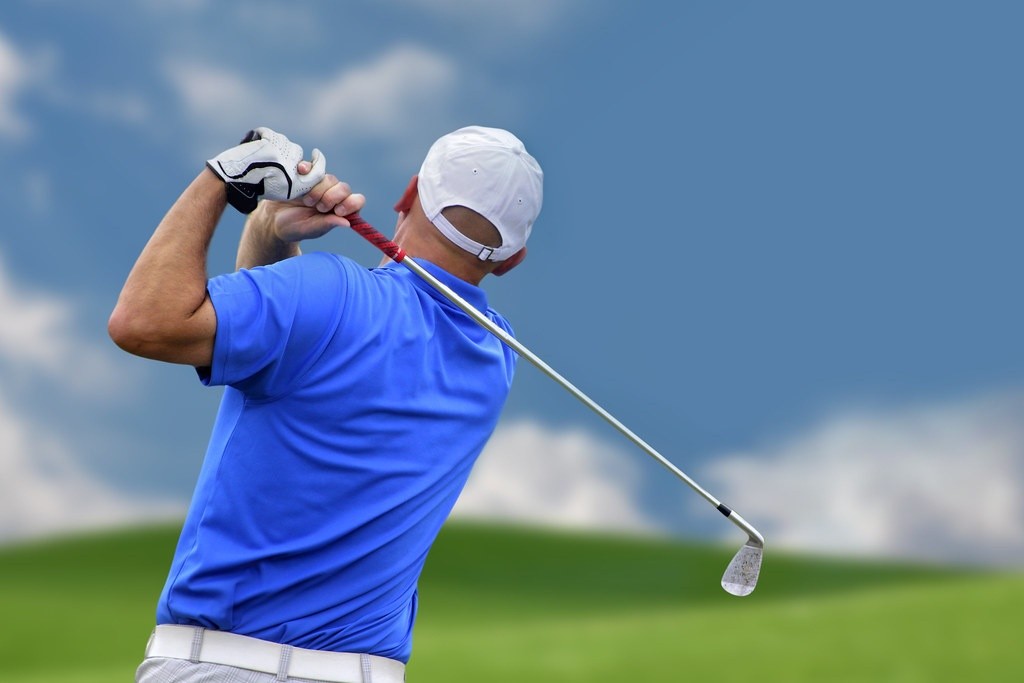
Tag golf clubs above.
[240,130,766,598]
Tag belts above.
[148,624,405,683]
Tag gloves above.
[206,127,326,214]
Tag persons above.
[108,126,544,683]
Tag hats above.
[417,126,543,262]
